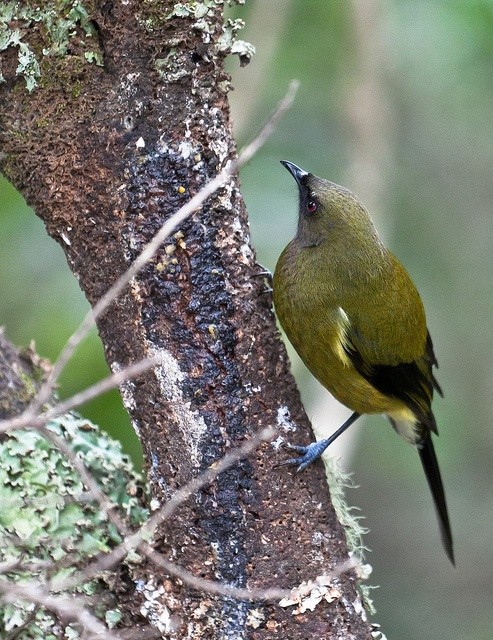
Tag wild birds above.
[249,159,457,569]
